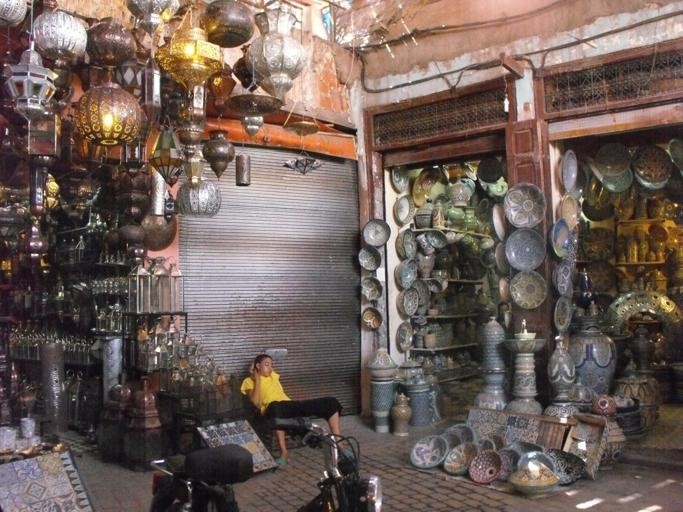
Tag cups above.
[21,417,35,438]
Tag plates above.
[391,168,406,193]
[549,149,578,331]
[595,140,683,192]
[358,219,391,330]
[397,321,412,352]
[491,181,546,328]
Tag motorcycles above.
[148,419,383,512]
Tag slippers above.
[339,450,352,457]
[279,456,287,465]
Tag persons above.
[240,355,354,467]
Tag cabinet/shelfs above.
[123,311,232,457]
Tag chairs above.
[242,397,302,450]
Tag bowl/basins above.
[396,288,419,317]
[395,229,416,259]
[410,424,586,500]
[427,230,448,249]
[394,258,417,288]
[393,194,415,227]
